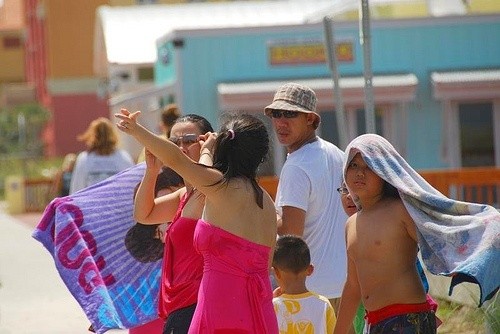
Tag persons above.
[264,83,344,316]
[133,114,218,334]
[138,104,180,165]
[56,153,77,197]
[340,174,429,334]
[114,108,279,334]
[333,134,499,333]
[125,165,185,264]
[272,235,337,334]
[69,118,134,198]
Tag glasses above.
[167,133,201,149]
[271,110,301,118]
[337,186,348,195]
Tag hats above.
[263,82,321,129]
